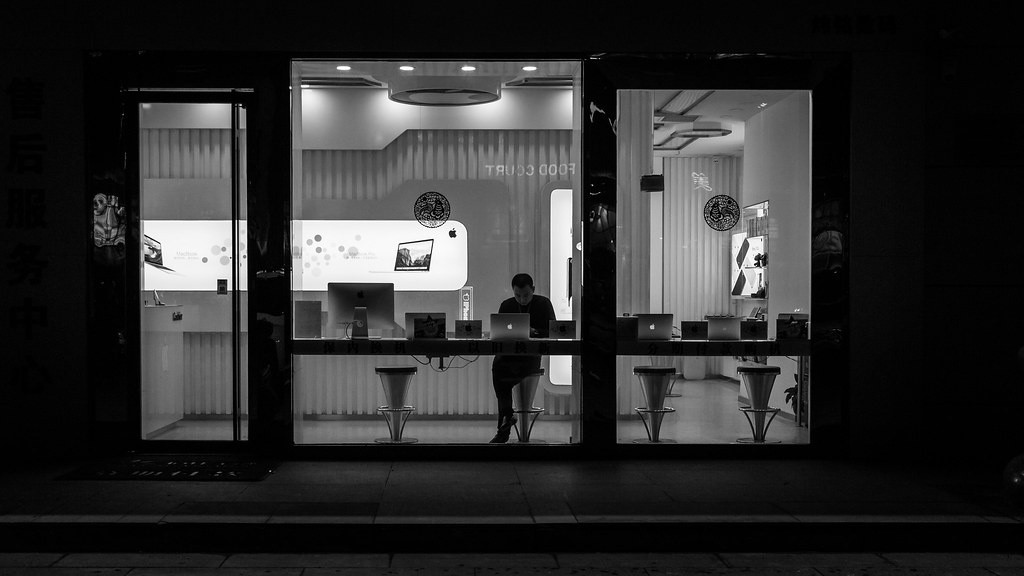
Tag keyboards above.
[346,336,381,339]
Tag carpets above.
[52,455,282,481]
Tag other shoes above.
[500,414,517,431]
[489,432,509,443]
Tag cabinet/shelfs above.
[731,232,768,339]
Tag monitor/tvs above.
[327,283,394,340]
[394,239,434,271]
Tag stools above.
[375,367,417,443]
[632,365,676,445]
[509,368,546,444]
[736,365,782,443]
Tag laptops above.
[489,313,530,340]
[549,320,576,339]
[404,313,445,340]
[707,316,740,340]
[633,313,673,340]
[775,318,808,341]
[740,321,768,340]
[681,321,708,340]
[617,316,638,342]
[143,234,175,271]
[748,306,761,317]
[455,320,482,339]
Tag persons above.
[488,272,556,443]
